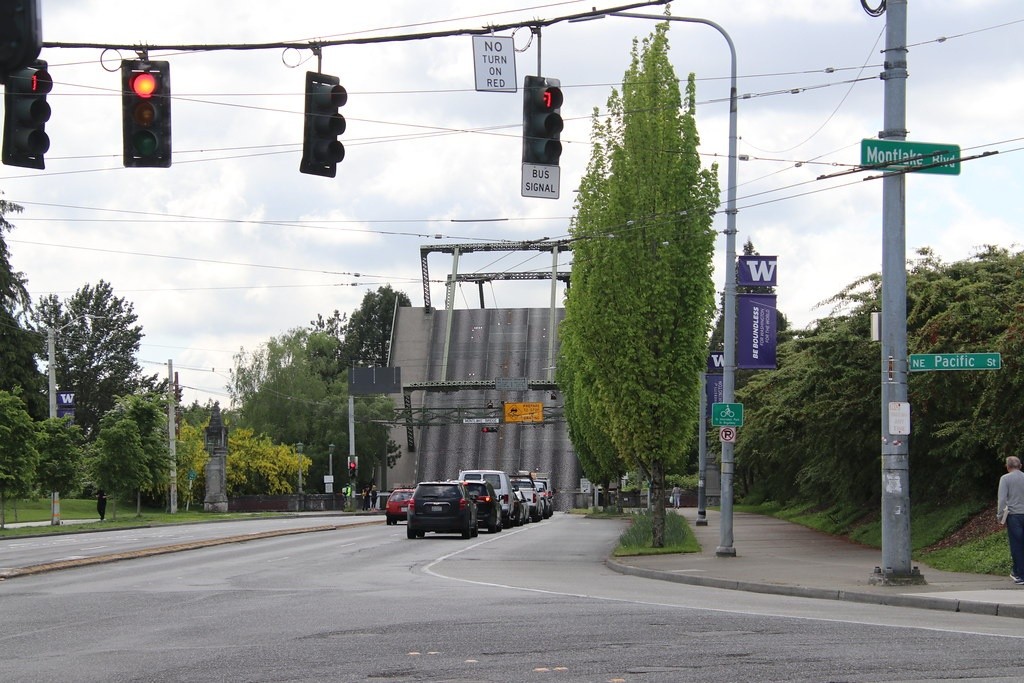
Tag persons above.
[363,484,377,511]
[342,483,351,507]
[996,456,1024,584]
[95,486,106,520]
[671,484,680,508]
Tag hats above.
[346,483,349,486]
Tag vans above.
[459,469,516,528]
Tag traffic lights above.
[122,59,174,169]
[301,68,348,177]
[1,57,53,170]
[348,456,358,478]
[481,427,499,433]
[523,77,563,169]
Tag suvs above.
[509,479,544,522]
[406,481,479,539]
[463,480,505,533]
[510,472,554,519]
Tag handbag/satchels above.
[669,495,673,503]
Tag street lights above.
[46,315,105,524]
[296,441,305,492]
[328,444,336,476]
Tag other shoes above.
[1010,573,1024,585]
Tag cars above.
[511,492,525,526]
[515,491,532,524]
[385,488,415,525]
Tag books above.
[1001,505,1009,525]
